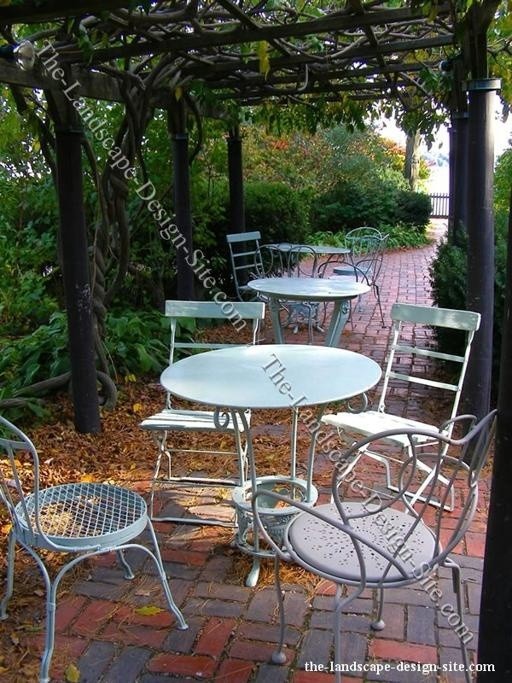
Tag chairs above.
[0,224,496,683]
